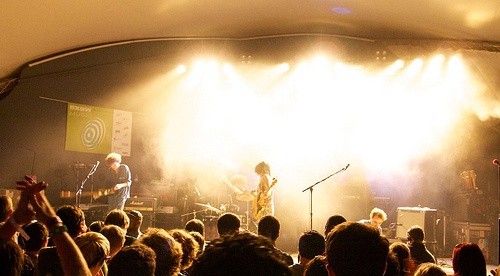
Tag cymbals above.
[194,202,221,214]
[236,191,256,201]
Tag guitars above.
[250,179,278,217]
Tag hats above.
[126,210,143,221]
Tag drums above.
[221,204,240,212]
[203,216,220,241]
[235,214,248,231]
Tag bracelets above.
[46,216,63,228]
[8,210,30,241]
[51,224,68,241]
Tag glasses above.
[455,243,462,249]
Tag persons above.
[358,207,388,235]
[253,162,275,218]
[105,153,133,214]
[0,174,500,276]
